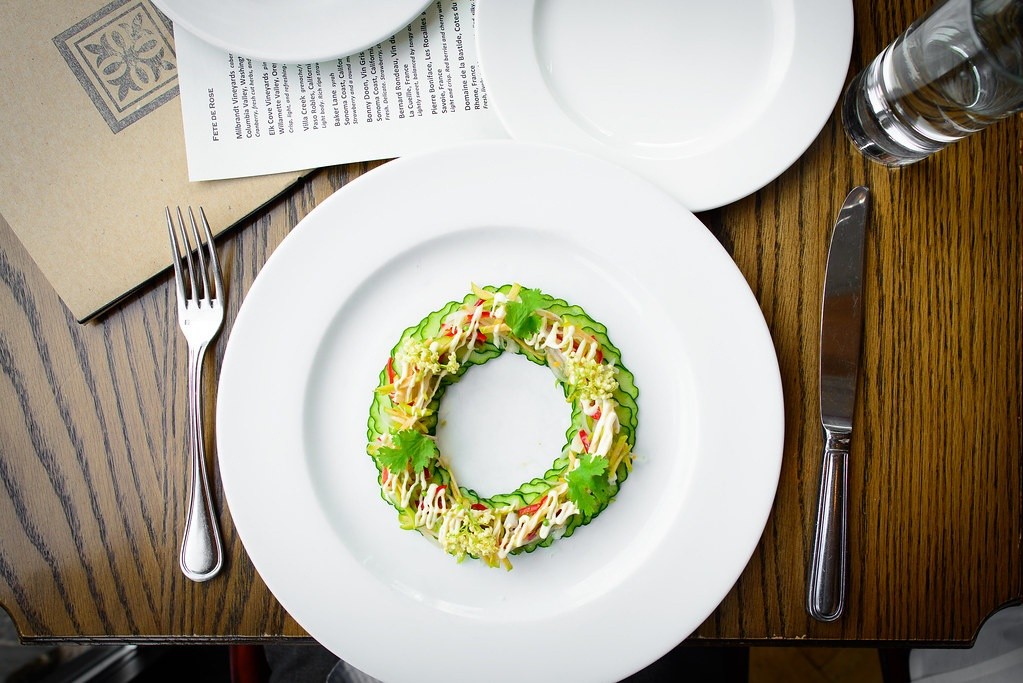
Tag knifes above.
[807,185,873,621]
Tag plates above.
[151,0,436,66]
[474,0,858,212]
[214,139,785,682]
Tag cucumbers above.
[367,283,639,559]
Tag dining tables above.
[0,1,1023,683]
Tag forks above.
[164,205,226,581]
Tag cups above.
[840,0,1023,167]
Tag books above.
[1,1,335,329]
